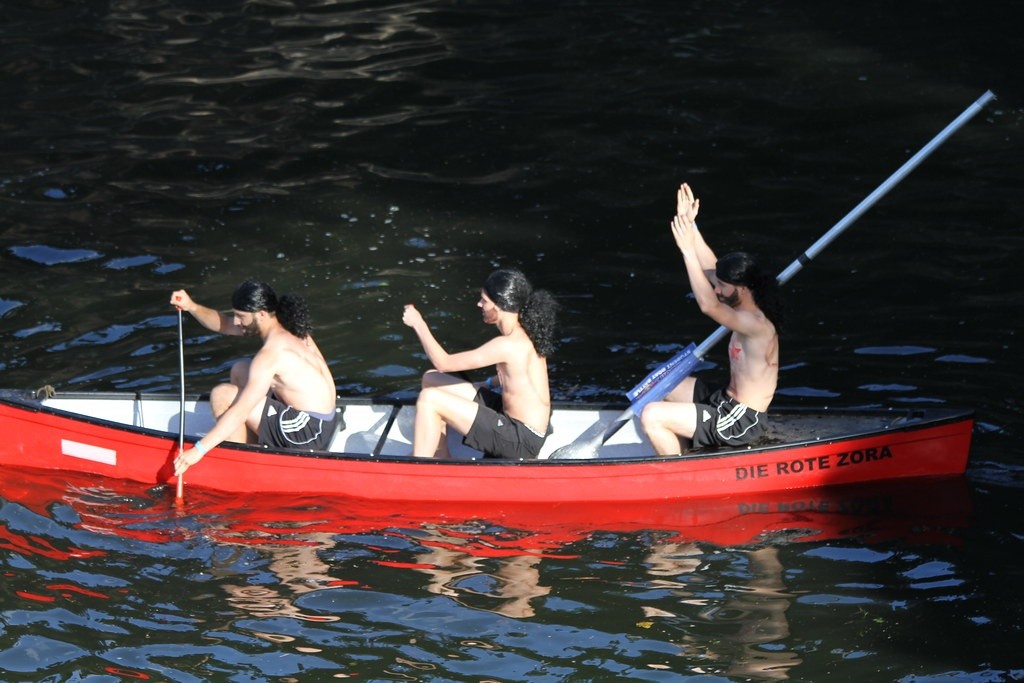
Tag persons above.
[641,182,781,456]
[402,270,558,459]
[170,280,338,479]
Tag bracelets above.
[487,377,495,389]
[196,441,206,454]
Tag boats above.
[0,387,975,526]
